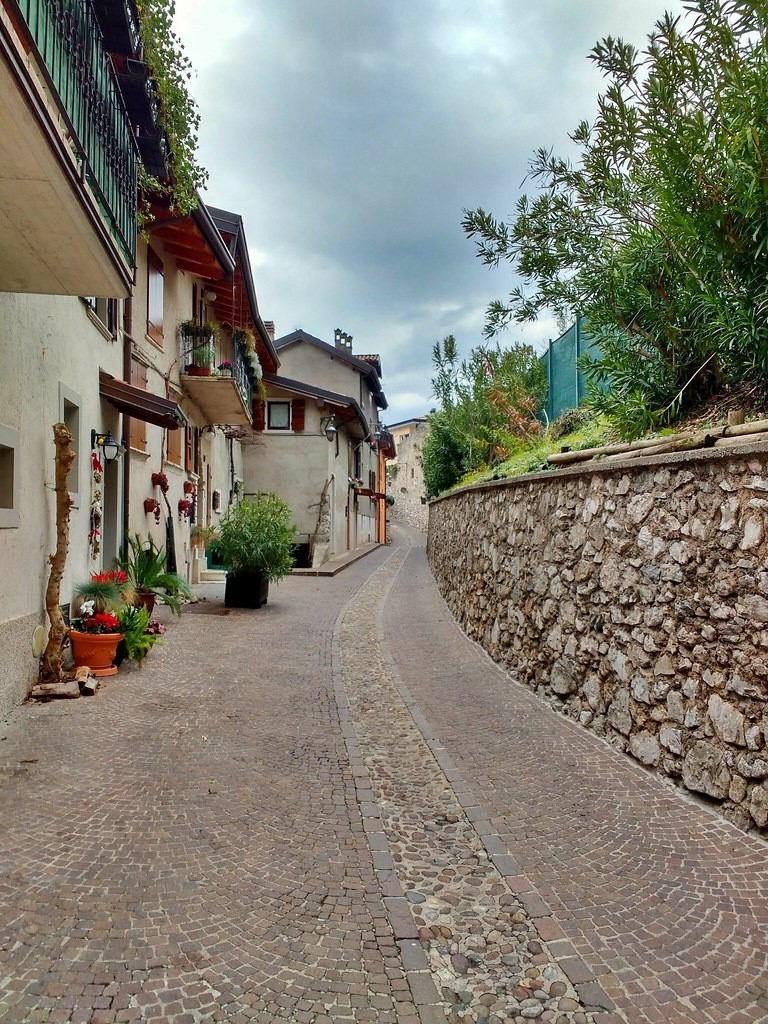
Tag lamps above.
[201,287,217,302]
[369,422,383,441]
[386,480,392,486]
[320,413,339,442]
[91,428,119,465]
[199,423,216,441]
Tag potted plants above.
[350,477,364,489]
[233,325,267,403]
[76,529,192,668]
[178,318,225,376]
[205,480,302,610]
[93,0,209,245]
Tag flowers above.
[217,360,238,371]
[371,441,378,451]
[68,600,122,634]
[91,572,128,584]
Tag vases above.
[68,629,125,677]
[222,368,232,376]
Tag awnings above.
[99,372,188,431]
